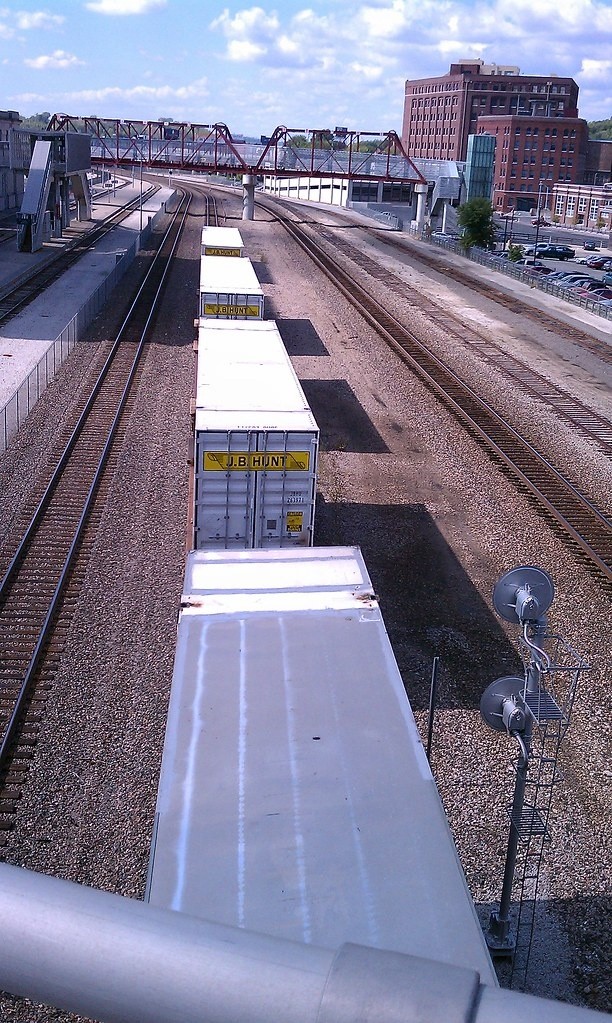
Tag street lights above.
[533,180,543,263]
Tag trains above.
[142,225,500,990]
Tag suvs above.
[583,241,596,250]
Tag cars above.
[429,226,462,242]
[530,218,551,228]
[486,241,612,306]
